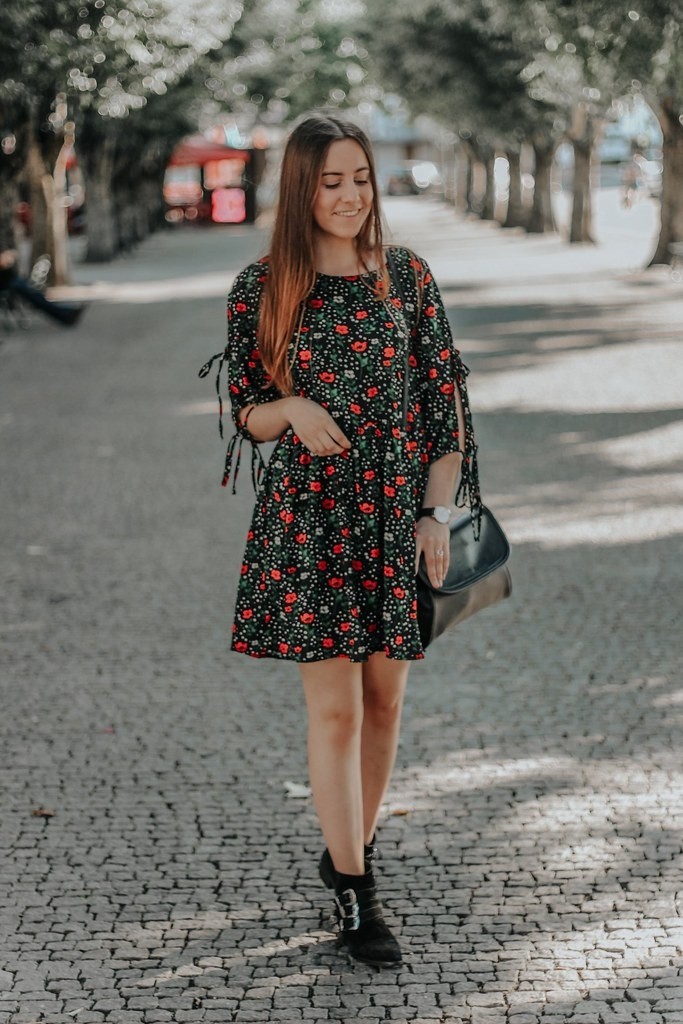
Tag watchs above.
[416,506,453,524]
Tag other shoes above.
[52,300,91,329]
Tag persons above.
[0,250,85,327]
[198,117,484,969]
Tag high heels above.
[319,832,403,970]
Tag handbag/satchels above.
[417,505,513,649]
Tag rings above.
[435,550,444,555]
